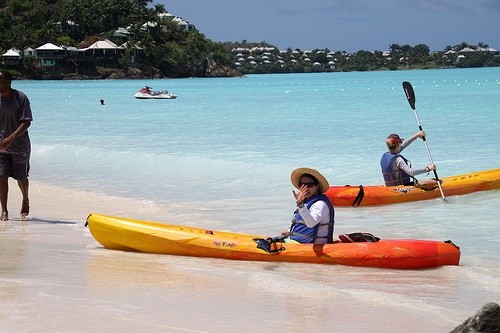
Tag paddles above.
[252,232,287,253]
[402,81,449,203]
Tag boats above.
[323,167,500,207]
[87,213,461,269]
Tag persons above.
[0,70,33,221]
[380,130,437,188]
[145,87,151,93]
[282,167,334,245]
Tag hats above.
[386,134,404,150]
[291,167,329,193]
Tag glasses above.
[298,182,315,188]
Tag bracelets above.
[427,167,431,171]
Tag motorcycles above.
[134,85,178,99]
